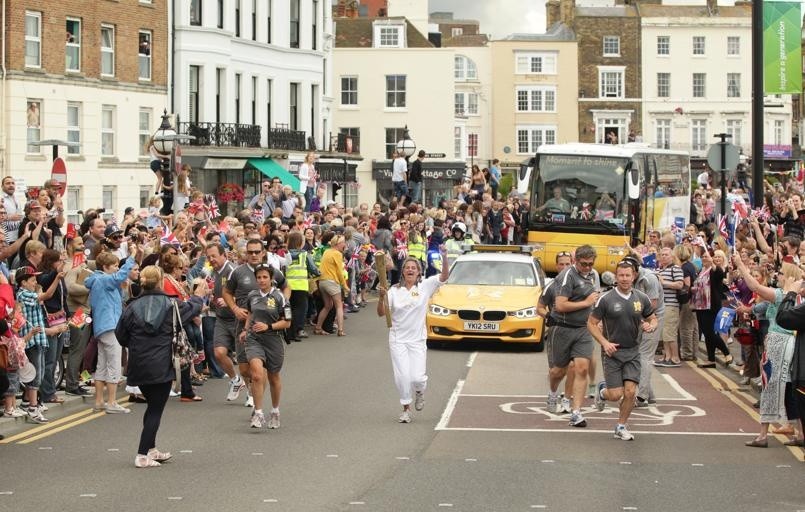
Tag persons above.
[1,150,531,429]
[114,266,206,467]
[530,163,804,447]
[148,135,172,198]
[605,130,643,144]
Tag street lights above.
[154,110,176,226]
[396,123,415,187]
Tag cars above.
[426,243,550,349]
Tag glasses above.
[113,236,122,240]
[246,226,255,229]
[698,234,704,238]
[400,222,406,225]
[247,250,261,255]
[778,271,784,276]
[713,242,718,244]
[282,229,290,232]
[557,252,633,267]
[779,200,785,203]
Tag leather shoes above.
[288,338,301,342]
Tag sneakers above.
[653,336,734,368]
[0,402,49,424]
[227,377,280,428]
[415,393,425,411]
[129,396,136,402]
[739,378,805,447]
[136,398,147,403]
[169,391,178,397]
[398,411,411,423]
[65,386,131,414]
[547,380,656,440]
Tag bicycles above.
[17,350,65,396]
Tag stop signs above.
[175,144,182,176]
[51,156,67,198]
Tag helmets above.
[451,221,467,238]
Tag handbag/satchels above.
[169,300,200,370]
[8,321,29,369]
[0,337,17,372]
[42,280,68,338]
[733,321,761,345]
[671,266,690,305]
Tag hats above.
[104,224,123,236]
[44,178,62,189]
[15,266,43,279]
[24,200,41,210]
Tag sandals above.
[338,330,346,336]
[147,449,172,461]
[43,398,64,403]
[313,328,330,335]
[181,396,202,401]
[135,456,161,467]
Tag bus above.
[520,142,691,276]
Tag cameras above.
[132,234,136,241]
[192,283,199,292]
[273,245,282,250]
[292,191,296,194]
[47,210,56,218]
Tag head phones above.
[621,257,640,272]
